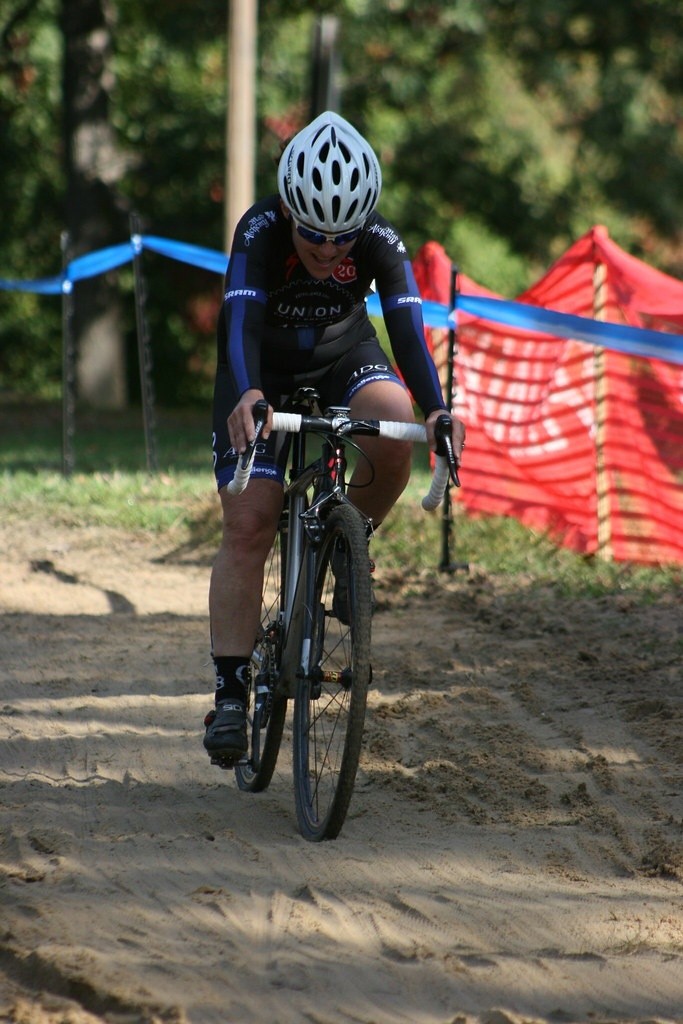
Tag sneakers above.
[204,701,250,759]
[334,577,378,627]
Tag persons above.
[202,110,467,767]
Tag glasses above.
[291,213,365,247]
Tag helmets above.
[277,109,381,236]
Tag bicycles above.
[231,386,462,842]
